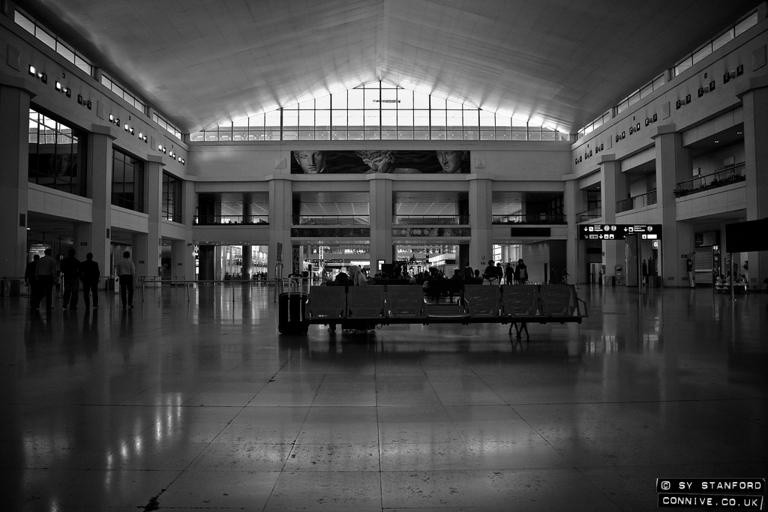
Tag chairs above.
[305,286,589,342]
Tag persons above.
[59,247,80,312]
[80,253,100,309]
[687,256,696,289]
[513,258,528,285]
[117,251,135,309]
[642,259,648,287]
[40,249,58,309]
[292,152,333,174]
[25,254,54,321]
[356,150,422,174]
[744,261,749,282]
[435,150,470,175]
[224,271,266,282]
[497,263,503,284]
[322,264,484,293]
[505,262,515,284]
[485,260,497,285]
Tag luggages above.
[278,273,309,333]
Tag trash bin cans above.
[647,272,658,287]
[10,279,24,297]
[109,276,119,294]
